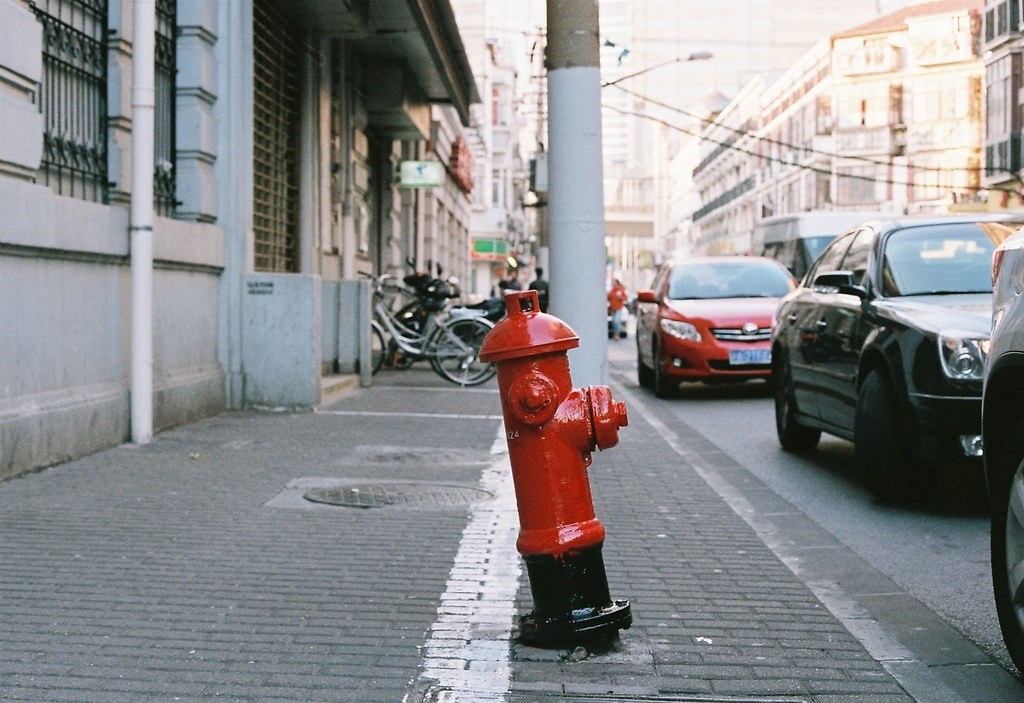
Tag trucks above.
[754,210,889,286]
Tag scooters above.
[384,257,510,370]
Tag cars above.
[634,256,800,396]
[768,212,1024,507]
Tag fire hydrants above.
[474,285,639,649]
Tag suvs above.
[977,222,1024,671]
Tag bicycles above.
[358,270,508,387]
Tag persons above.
[499,266,522,293]
[606,279,629,340]
[528,266,549,313]
